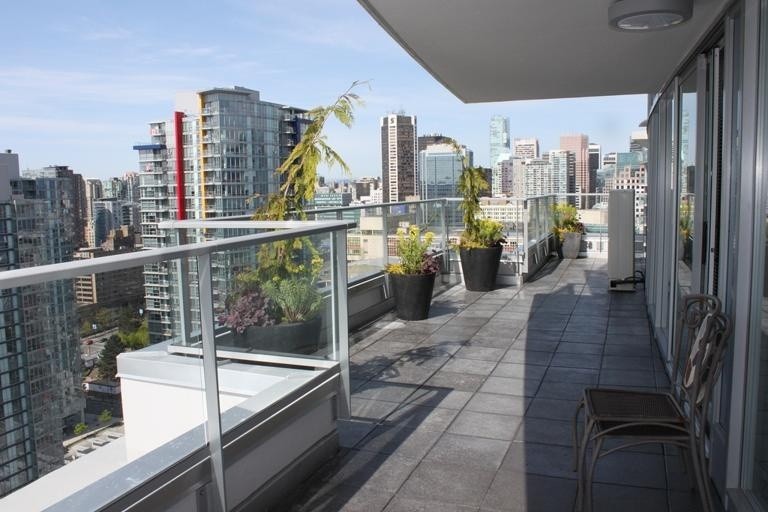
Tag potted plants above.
[441,137,516,291]
[381,223,444,320]
[547,202,586,259]
[215,76,379,354]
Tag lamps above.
[607,1,693,33]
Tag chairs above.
[569,292,731,511]
